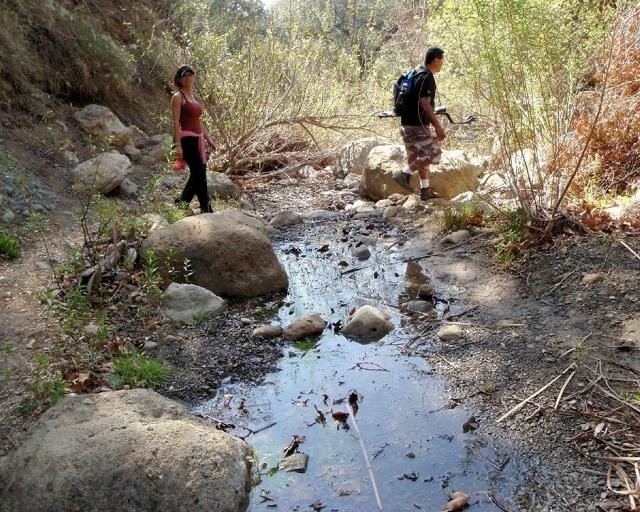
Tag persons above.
[392,46,446,201]
[170,65,216,214]
